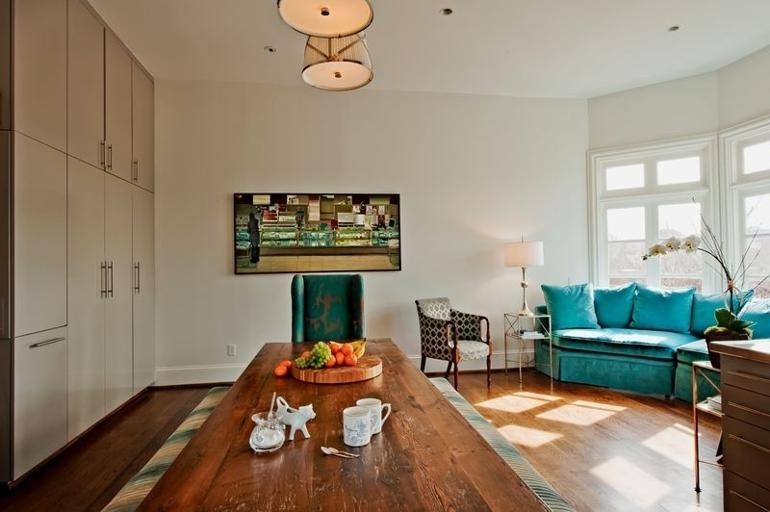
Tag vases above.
[702,331,751,368]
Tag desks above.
[102,338,574,511]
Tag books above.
[519,330,544,339]
[707,395,722,411]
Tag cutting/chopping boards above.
[291,354,382,384]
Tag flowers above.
[641,193,770,336]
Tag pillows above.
[630,281,697,334]
[692,289,754,337]
[735,294,770,339]
[540,283,600,331]
[593,282,639,329]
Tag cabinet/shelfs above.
[69,155,134,448]
[708,340,770,512]
[1,130,70,342]
[503,314,553,385]
[134,51,161,193]
[0,1,68,154]
[133,183,156,398]
[3,326,69,489]
[69,1,133,184]
[691,360,723,492]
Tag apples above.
[344,354,357,366]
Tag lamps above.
[276,0,375,37]
[301,34,374,91]
[505,233,546,316]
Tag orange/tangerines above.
[330,343,338,352]
[342,343,353,355]
[302,350,310,358]
[336,352,344,365]
[273,366,288,377]
[279,360,292,366]
[326,355,336,368]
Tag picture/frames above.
[233,192,402,274]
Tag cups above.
[342,407,380,448]
[356,398,393,436]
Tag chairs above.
[415,296,492,390]
[292,273,366,344]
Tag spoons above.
[321,446,354,460]
[328,447,361,458]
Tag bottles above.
[249,412,286,455]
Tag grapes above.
[295,341,332,369]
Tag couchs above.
[535,283,770,403]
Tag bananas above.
[328,337,367,360]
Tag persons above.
[246,212,261,263]
